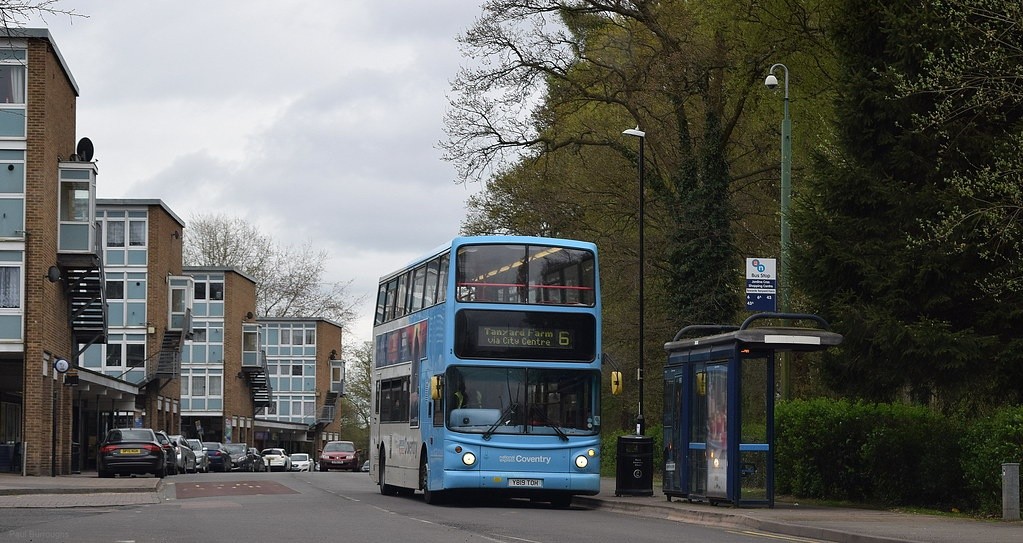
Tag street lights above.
[762,60,795,402]
[622,125,649,435]
[51,359,70,477]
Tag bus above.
[369,235,623,510]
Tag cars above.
[186,439,209,473]
[250,447,261,473]
[362,460,370,471]
[99,428,166,478]
[168,434,197,474]
[289,453,315,473]
[223,443,255,472]
[202,441,232,472]
[315,460,320,471]
[155,430,178,475]
[260,448,291,472]
[318,441,360,472]
